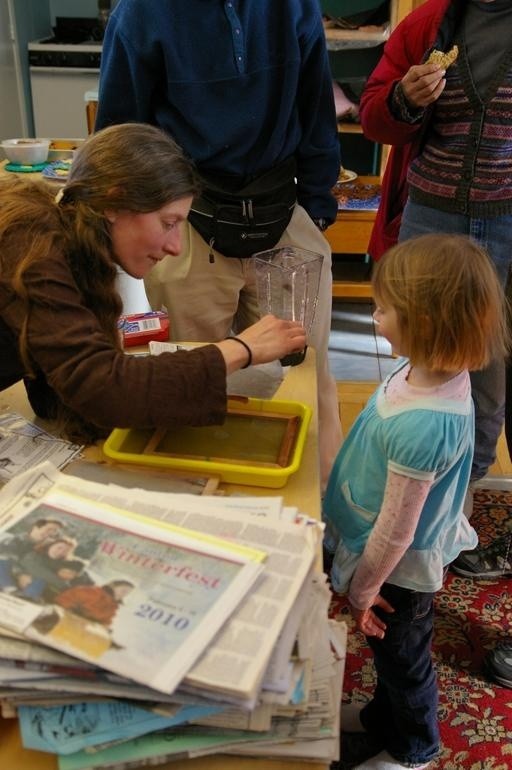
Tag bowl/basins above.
[1,138,52,165]
[324,19,336,29]
[49,138,87,162]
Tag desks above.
[1,336,341,768]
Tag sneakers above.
[487,643,512,690]
[451,538,511,581]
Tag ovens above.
[29,65,102,139]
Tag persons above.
[95,0,347,457]
[321,230,510,770]
[362,0,511,520]
[2,119,309,435]
[450,257,511,686]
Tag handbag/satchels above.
[188,178,299,259]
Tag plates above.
[42,158,72,180]
[335,170,358,187]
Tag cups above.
[253,243,327,369]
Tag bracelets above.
[227,332,256,375]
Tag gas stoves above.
[28,31,104,48]
